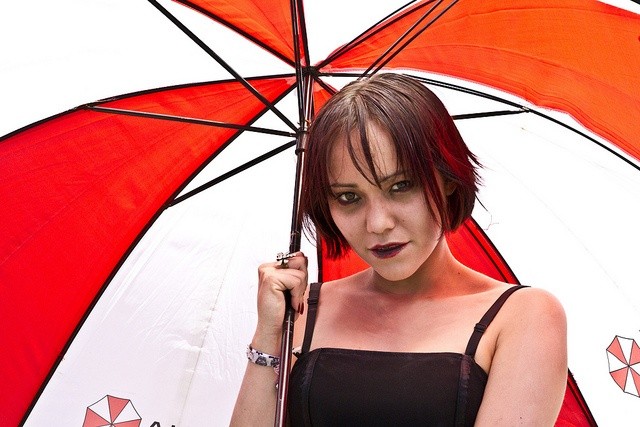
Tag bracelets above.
[245,343,281,368]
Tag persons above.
[228,71,568,425]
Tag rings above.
[276,251,297,268]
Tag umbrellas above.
[2,0,640,427]
